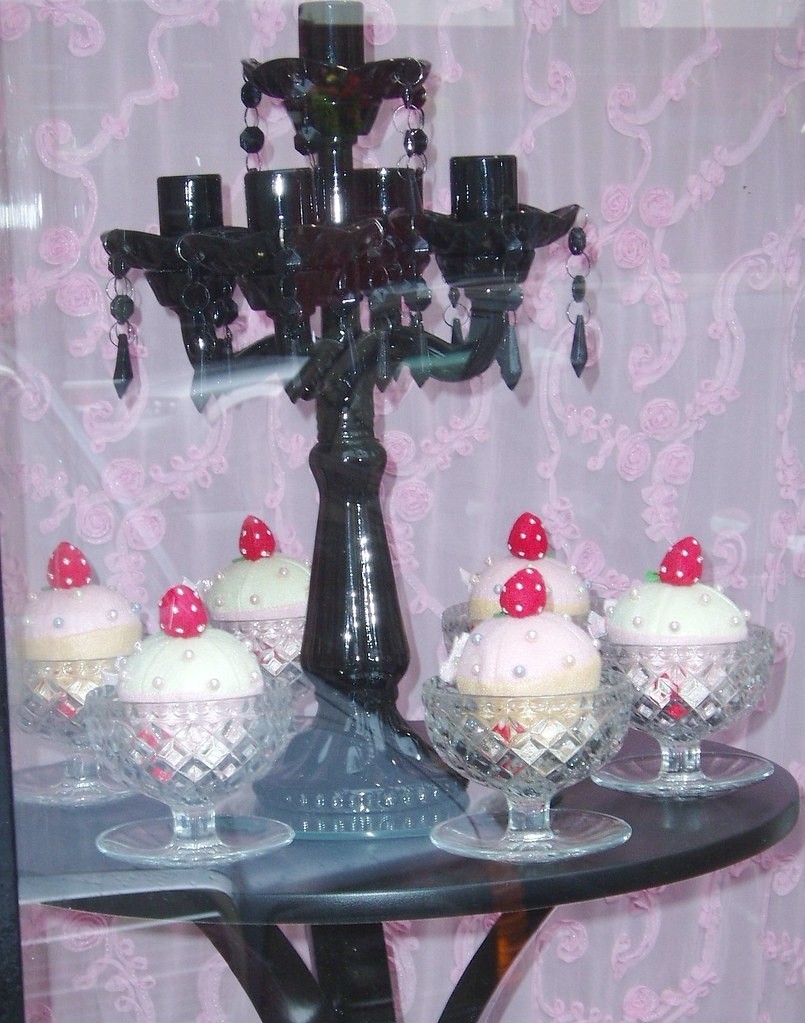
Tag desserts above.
[453,511,748,794]
[15,515,313,810]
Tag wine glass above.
[15,656,139,810]
[591,623,777,799]
[421,599,634,863]
[82,618,312,867]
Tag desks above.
[13,719,798,1022]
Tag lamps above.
[97,0,591,814]
